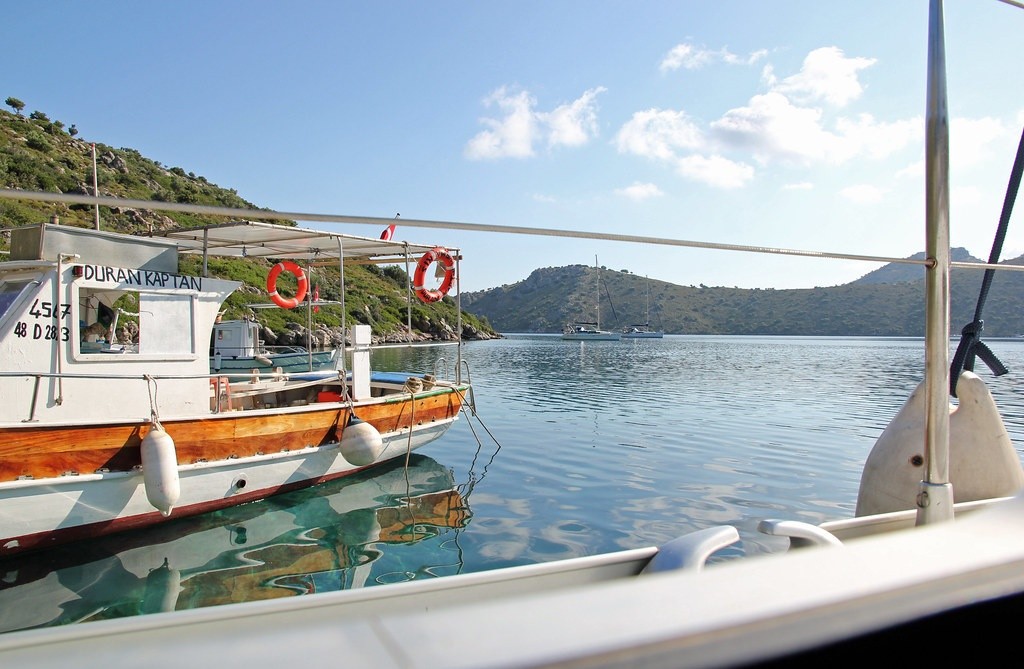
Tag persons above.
[210,314,223,357]
[82,314,111,342]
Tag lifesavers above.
[413,247,456,303]
[267,261,307,309]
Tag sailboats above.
[620,273,665,338]
[561,254,623,340]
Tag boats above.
[208,317,342,381]
[0,140,479,559]
